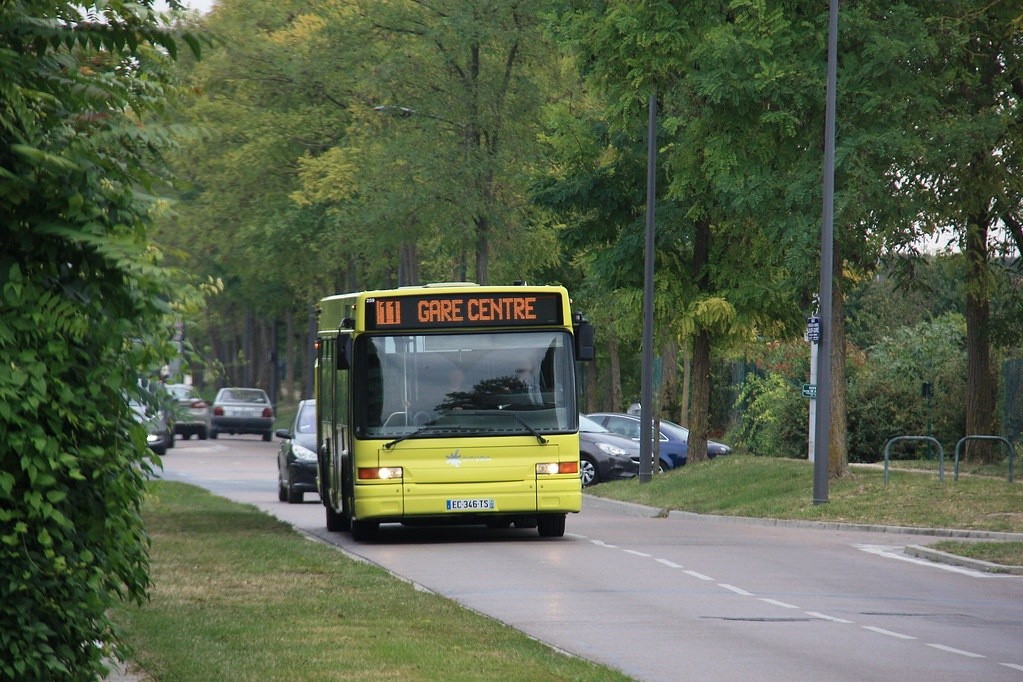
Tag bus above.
[316,277,595,540]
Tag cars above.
[583,413,730,474]
[576,408,654,488]
[165,383,207,440]
[116,377,170,457]
[208,385,273,441]
[275,399,323,503]
